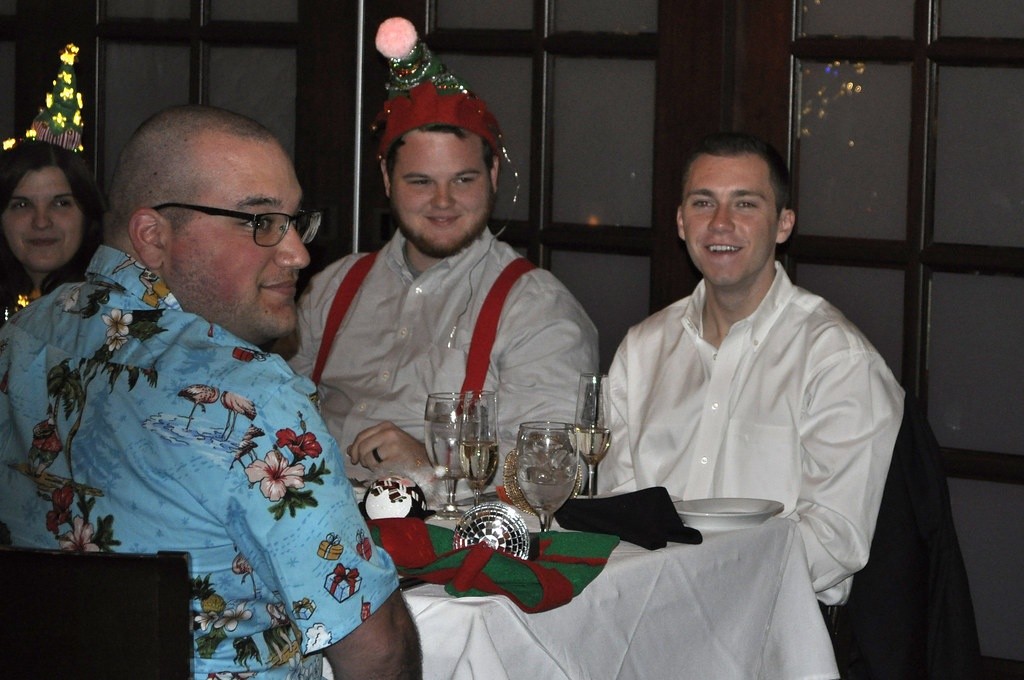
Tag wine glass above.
[573,372,614,499]
[459,388,500,507]
[424,392,469,519]
[516,421,581,532]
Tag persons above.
[592,131,908,634]
[0,106,423,680]
[257,17,600,499]
[0,37,106,327]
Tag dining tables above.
[336,466,847,680]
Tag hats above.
[371,81,504,160]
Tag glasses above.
[152,202,322,245]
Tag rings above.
[372,448,383,465]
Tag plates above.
[674,497,785,530]
